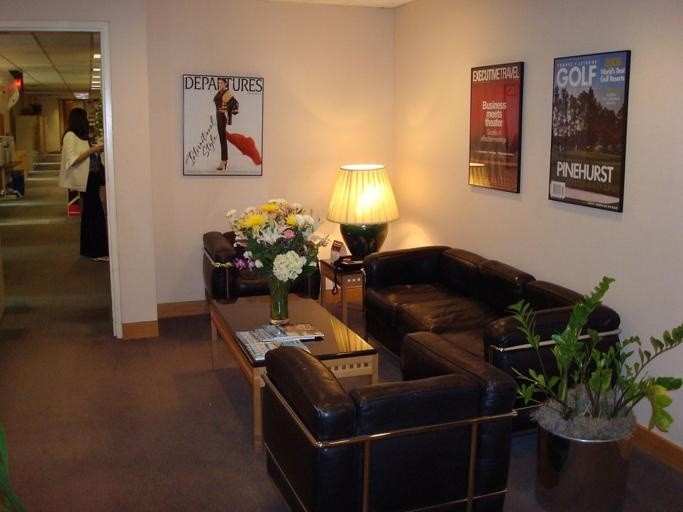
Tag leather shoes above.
[89,254,109,261]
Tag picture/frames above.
[179,73,266,178]
[466,60,526,194]
[546,48,634,215]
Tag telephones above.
[334,255,365,271]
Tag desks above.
[315,255,364,328]
[505,430,683,511]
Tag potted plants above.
[504,274,683,512]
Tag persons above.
[57,107,110,263]
[212,78,239,171]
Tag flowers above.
[222,196,332,321]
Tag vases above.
[267,270,292,326]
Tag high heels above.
[216,161,227,170]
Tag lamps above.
[322,162,403,262]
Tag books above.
[234,321,324,367]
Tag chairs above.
[255,326,521,512]
[196,227,323,305]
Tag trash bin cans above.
[10,170,25,195]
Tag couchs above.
[358,242,624,433]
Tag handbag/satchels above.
[96,153,105,187]
